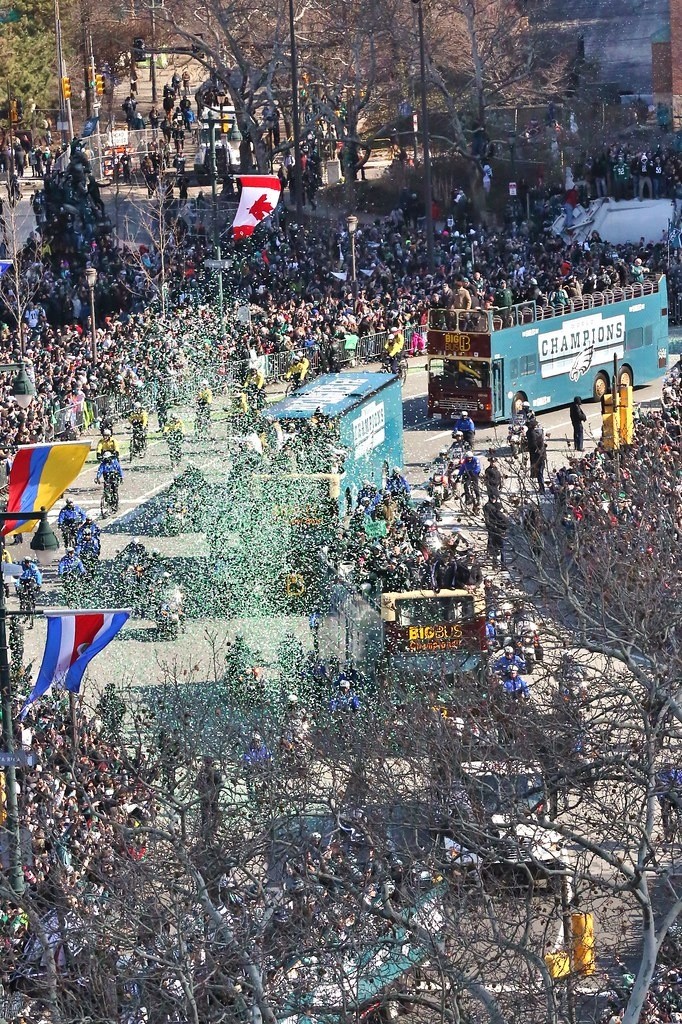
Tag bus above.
[66,793,444,1015]
[303,534,477,749]
[422,747,573,965]
[427,270,672,429]
[257,370,408,584]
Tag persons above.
[0,72,682,1023]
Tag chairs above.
[469,275,661,334]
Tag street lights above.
[0,505,60,908]
[345,214,358,315]
[85,269,98,366]
[0,361,35,409]
